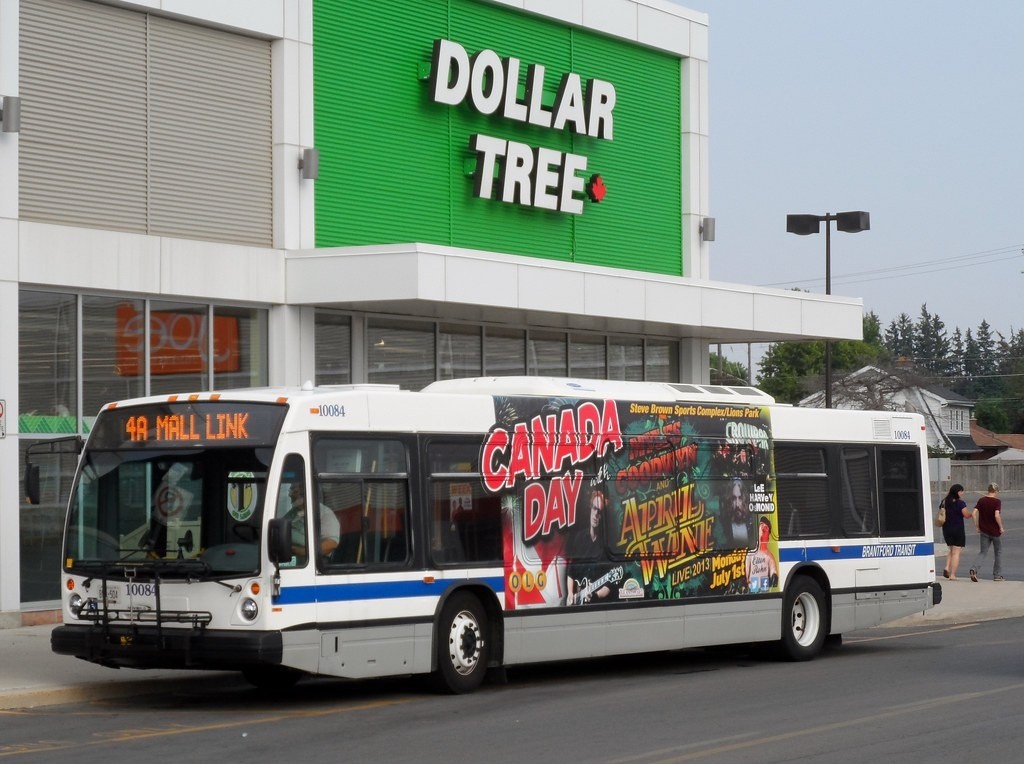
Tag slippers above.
[950,578,958,580]
[944,569,950,578]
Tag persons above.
[727,479,754,543]
[281,475,341,563]
[970,482,1006,582]
[565,491,621,606]
[744,516,777,593]
[939,484,972,580]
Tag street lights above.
[786,212,871,408]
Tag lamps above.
[699,218,715,242]
[298,148,319,178]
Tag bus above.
[24,374,943,695]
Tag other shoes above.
[994,576,1007,581]
[969,569,979,582]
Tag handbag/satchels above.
[935,499,946,527]
